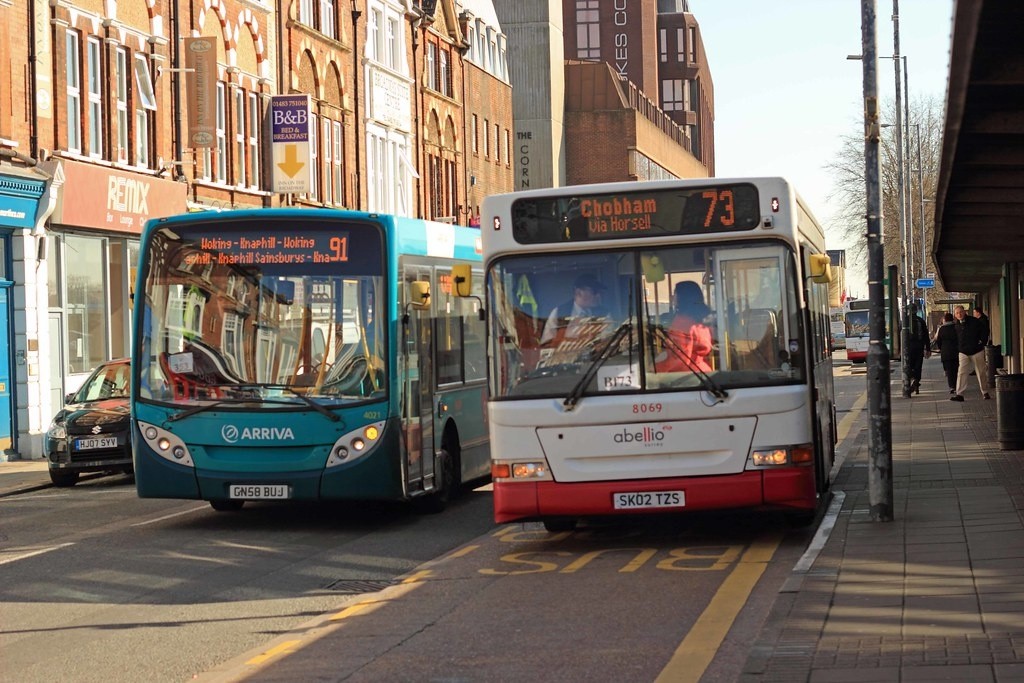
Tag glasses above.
[581,288,600,295]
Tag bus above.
[831,312,846,352]
[129,208,522,513]
[453,176,838,534]
[843,296,910,364]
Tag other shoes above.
[910,379,918,392]
[983,392,990,399]
[950,387,956,394]
[950,395,965,402]
[969,370,976,375]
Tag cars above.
[43,357,165,488]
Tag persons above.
[537,277,616,367]
[650,280,717,373]
[898,304,991,402]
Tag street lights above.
[879,122,929,328]
[846,54,912,399]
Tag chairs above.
[712,307,790,372]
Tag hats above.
[574,273,608,290]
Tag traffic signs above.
[269,94,316,194]
[915,278,935,289]
